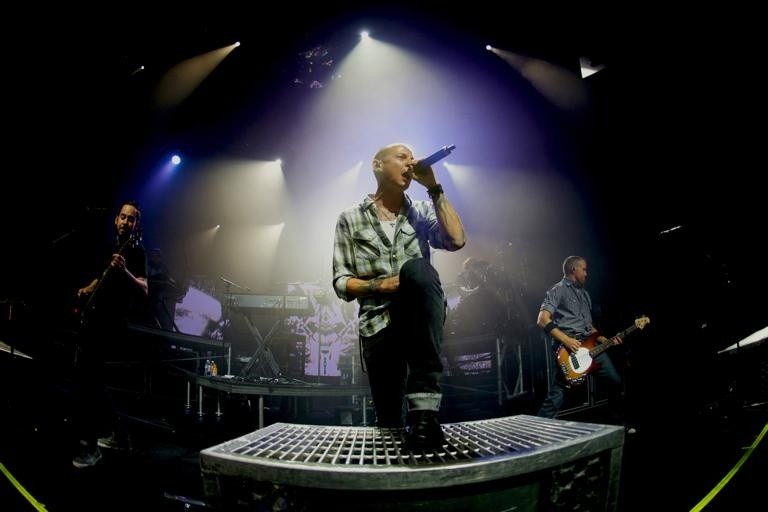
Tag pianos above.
[223,294,309,309]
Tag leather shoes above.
[402,410,444,449]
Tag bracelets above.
[425,184,442,198]
[543,322,556,334]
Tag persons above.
[534,254,639,435]
[332,142,466,446]
[68,199,156,469]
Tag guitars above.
[557,315,650,385]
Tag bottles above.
[204,360,218,377]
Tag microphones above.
[409,144,456,173]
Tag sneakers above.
[71,448,102,469]
[97,436,132,451]
